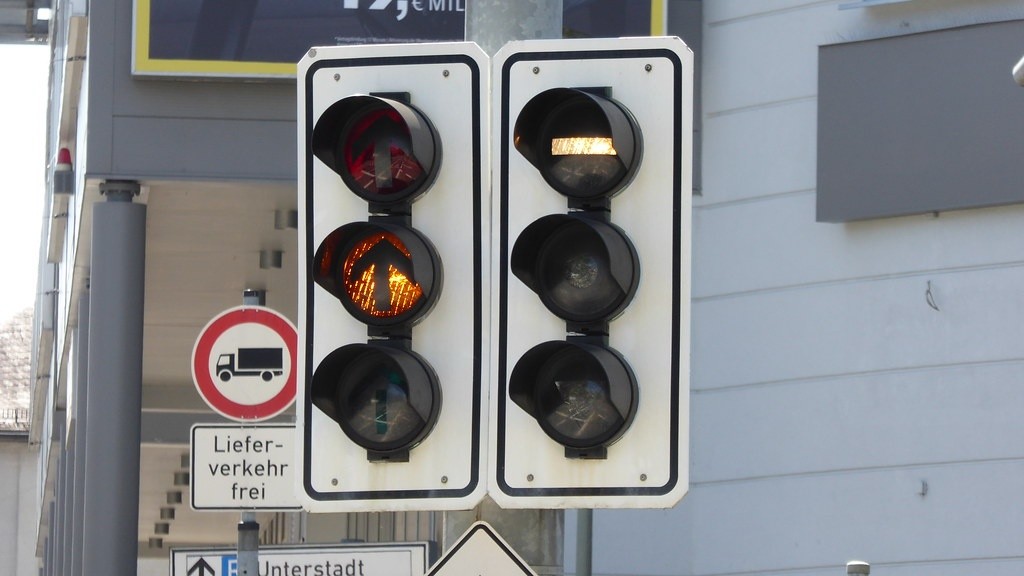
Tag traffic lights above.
[296,40,489,513]
[492,37,694,511]
